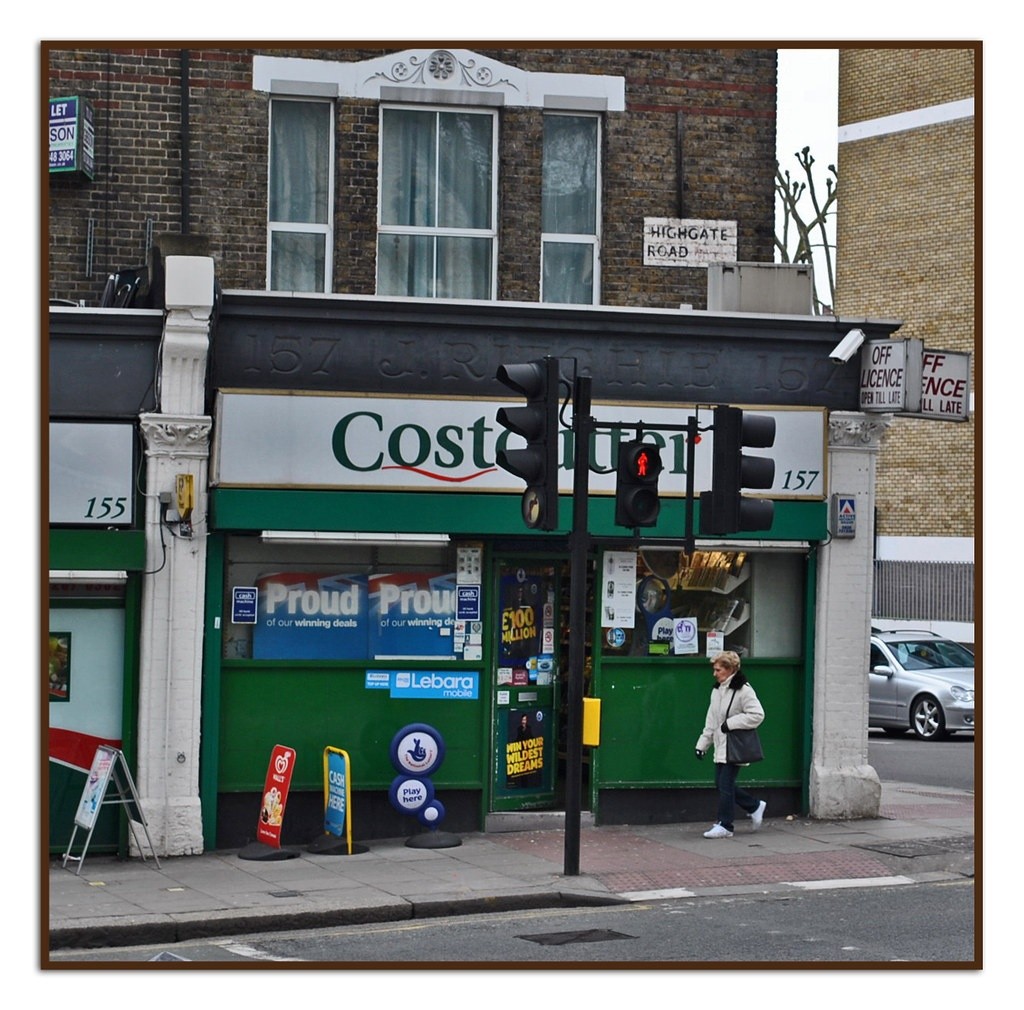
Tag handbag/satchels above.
[726,730,764,765]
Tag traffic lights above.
[496,355,560,531]
[713,405,776,535]
[615,441,662,528]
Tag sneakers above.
[750,800,766,830]
[704,821,733,838]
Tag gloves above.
[696,750,706,760]
[720,720,729,734]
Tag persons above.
[694,650,767,838]
[515,714,533,741]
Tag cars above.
[868,630,975,741]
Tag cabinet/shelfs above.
[557,563,751,763]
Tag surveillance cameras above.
[829,328,867,365]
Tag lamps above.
[259,531,451,546]
